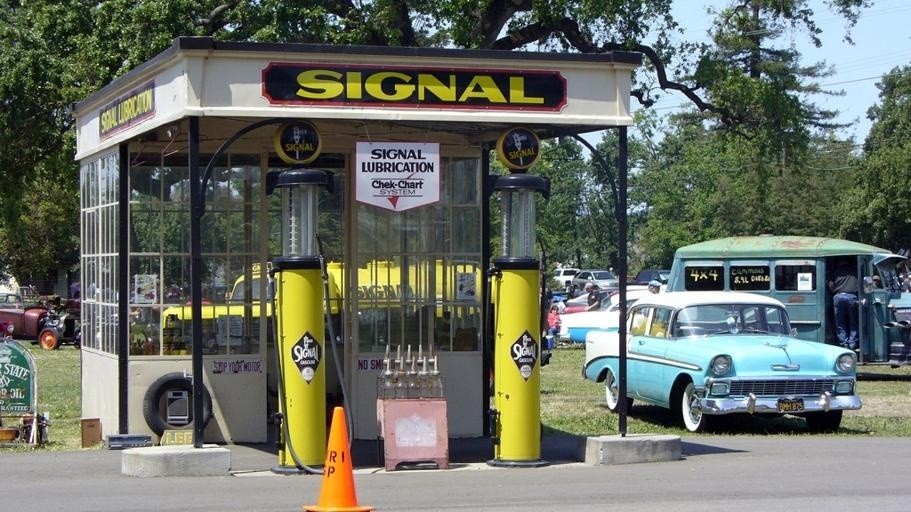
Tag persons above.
[826,262,858,350]
[874,271,911,292]
[638,279,662,300]
[547,305,561,348]
[584,283,601,312]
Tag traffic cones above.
[304,406,375,512]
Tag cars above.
[555,268,668,343]
[584,292,862,433]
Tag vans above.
[665,235,911,371]
